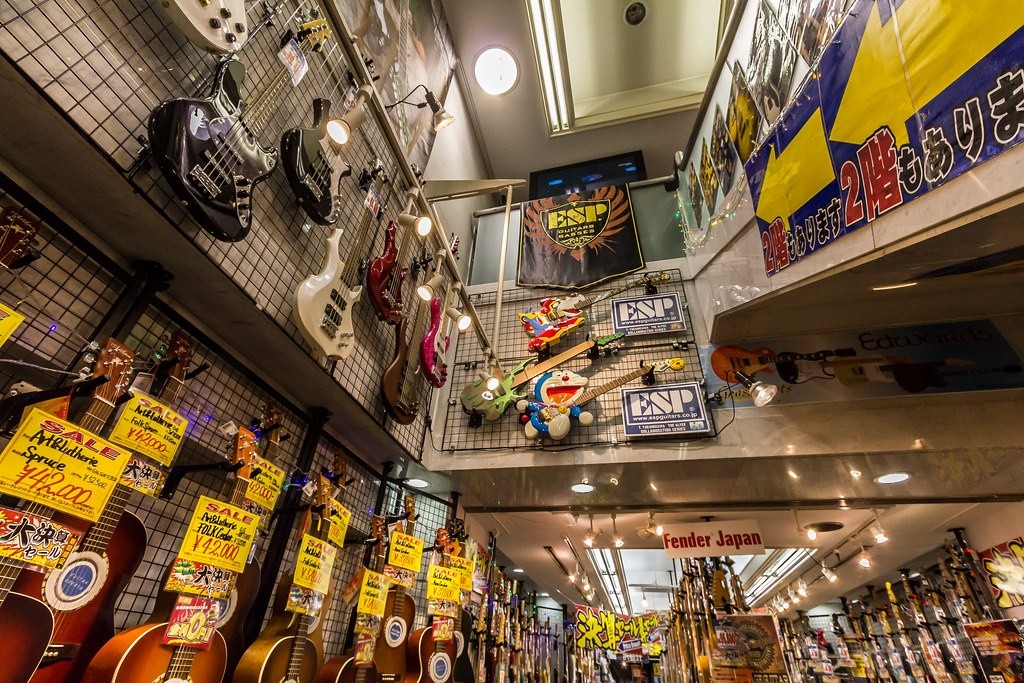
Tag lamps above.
[417,274,444,302]
[858,550,873,568]
[646,525,663,536]
[327,106,373,144]
[569,561,581,582]
[821,568,837,582]
[586,587,596,601]
[479,371,499,391]
[584,532,598,548]
[445,306,471,330]
[734,370,778,408]
[795,525,818,540]
[869,527,888,544]
[579,570,591,591]
[398,210,432,237]
[613,530,624,548]
[797,584,807,597]
[778,598,790,609]
[773,602,784,613]
[424,90,456,131]
[788,590,800,603]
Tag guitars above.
[516,271,671,353]
[158,0,251,59]
[380,235,442,425]
[365,175,433,326]
[0,206,1024,683]
[459,326,624,424]
[273,74,380,229]
[418,280,459,389]
[292,159,390,366]
[514,356,689,441]
[144,17,326,245]
